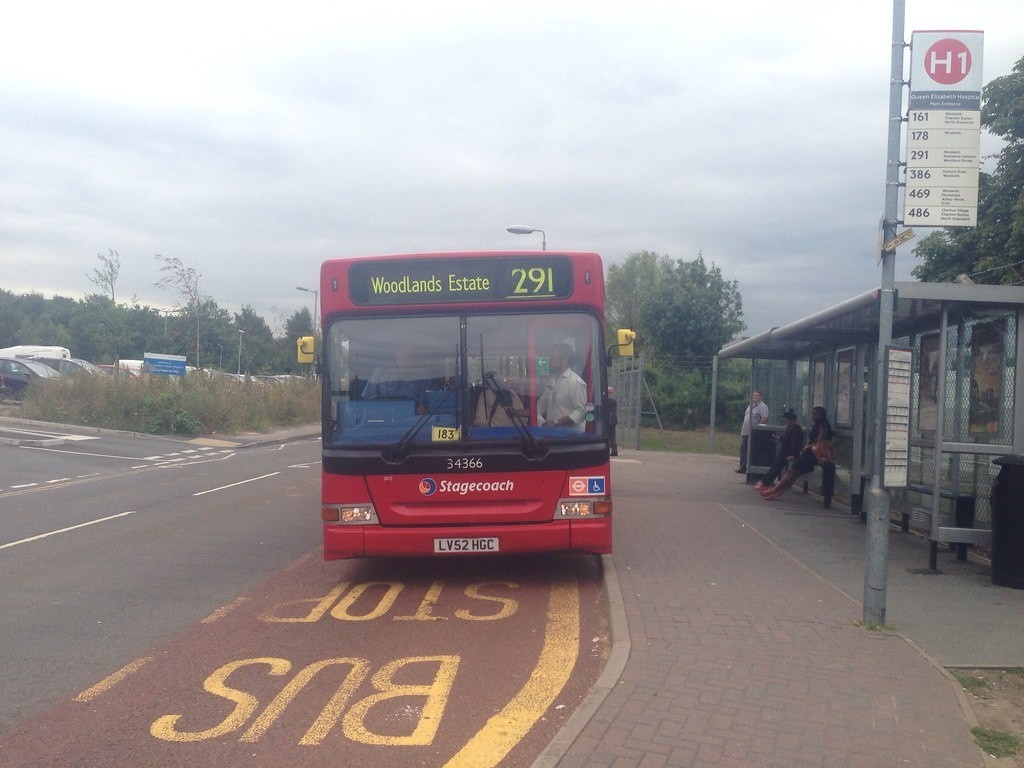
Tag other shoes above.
[610,453,618,456]
[760,489,777,499]
[754,481,765,490]
[735,469,745,474]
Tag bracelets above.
[554,418,560,427]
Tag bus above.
[296,250,635,559]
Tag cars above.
[0,339,319,417]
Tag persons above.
[364,333,439,413]
[734,388,772,472]
[604,387,619,458]
[473,370,529,428]
[761,406,833,500]
[531,342,588,431]
[752,408,804,490]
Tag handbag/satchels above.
[812,439,834,463]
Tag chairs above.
[433,377,528,428]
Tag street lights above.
[296,285,320,378]
[236,328,244,375]
[507,225,549,250]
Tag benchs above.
[804,463,834,508]
[860,475,975,559]
